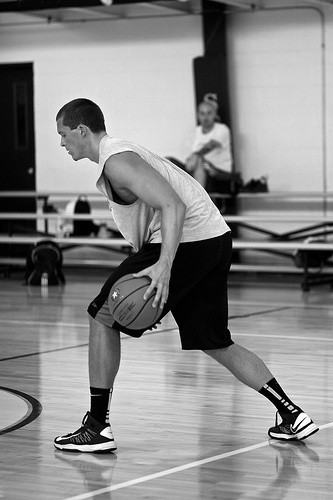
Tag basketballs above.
[108,273,164,330]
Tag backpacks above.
[64,195,95,236]
[25,240,66,285]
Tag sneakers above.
[268,410,320,440]
[53,411,117,453]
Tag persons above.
[53,99,319,452]
[166,92,233,189]
